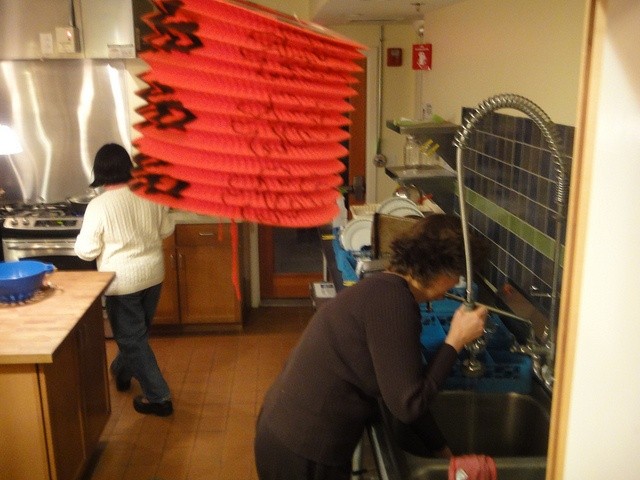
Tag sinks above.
[404,465,546,480]
[390,389,551,463]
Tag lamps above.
[0,123,23,157]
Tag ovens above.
[1,237,98,272]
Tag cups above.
[418,147,436,170]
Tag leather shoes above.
[111,361,131,391]
[133,395,173,416]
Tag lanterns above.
[124,1,368,299]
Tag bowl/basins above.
[0,260,57,303]
[69,193,99,216]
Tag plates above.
[386,206,425,217]
[340,218,373,252]
[378,197,417,215]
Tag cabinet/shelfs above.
[0,298,111,480]
[385,120,460,205]
[149,225,251,326]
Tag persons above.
[74,143,177,417]
[254,213,489,478]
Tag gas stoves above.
[0,201,83,233]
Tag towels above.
[449,455,497,480]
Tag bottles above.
[403,135,421,169]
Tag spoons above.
[393,187,411,200]
